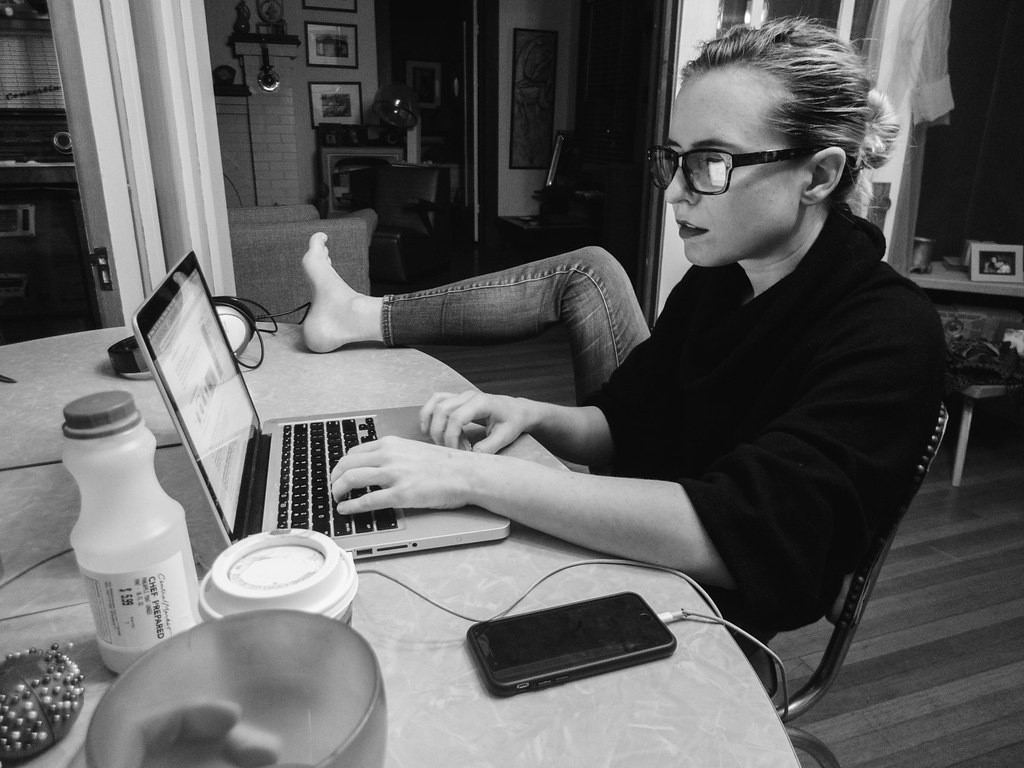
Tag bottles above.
[59,390,203,676]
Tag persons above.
[300,20,951,644]
[988,255,1011,274]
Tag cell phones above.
[469,592,677,696]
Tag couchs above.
[368,162,454,281]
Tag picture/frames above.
[302,0,357,13]
[319,148,404,219]
[308,81,363,128]
[969,242,1022,282]
[510,27,560,169]
[305,20,359,69]
[405,59,443,111]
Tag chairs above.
[755,409,955,768]
[228,203,371,324]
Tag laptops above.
[130,251,511,561]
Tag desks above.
[499,217,590,266]
[1,321,802,768]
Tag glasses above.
[646,144,828,195]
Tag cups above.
[85,609,388,768]
[195,525,360,636]
[912,235,935,269]
